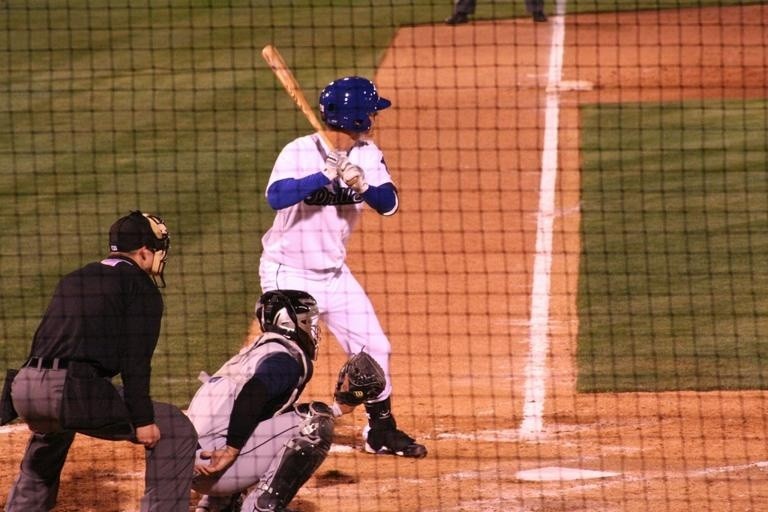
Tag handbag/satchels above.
[0,369,20,426]
[59,361,127,430]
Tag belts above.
[22,358,69,369]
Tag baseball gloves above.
[335,345,386,405]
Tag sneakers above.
[444,13,467,24]
[363,414,428,458]
[533,11,545,21]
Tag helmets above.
[318,75,392,132]
[109,212,170,252]
[255,289,319,332]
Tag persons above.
[185,290,334,512]
[444,0,548,24]
[4,212,201,512]
[260,75,428,459]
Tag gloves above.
[322,149,369,195]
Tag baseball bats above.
[263,44,358,186]
